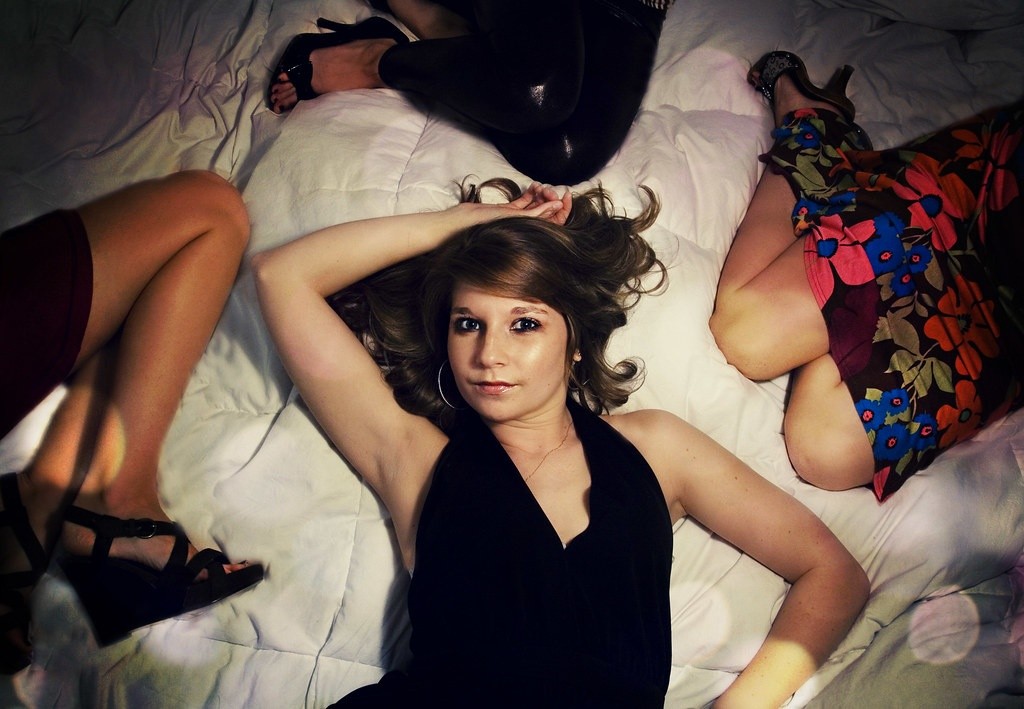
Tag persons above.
[709,50,1024,502]
[0,169,264,648]
[251,177,870,709]
[265,0,677,186]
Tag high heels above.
[748,50,857,121]
[0,473,49,678]
[266,15,410,114]
[53,507,264,648]
